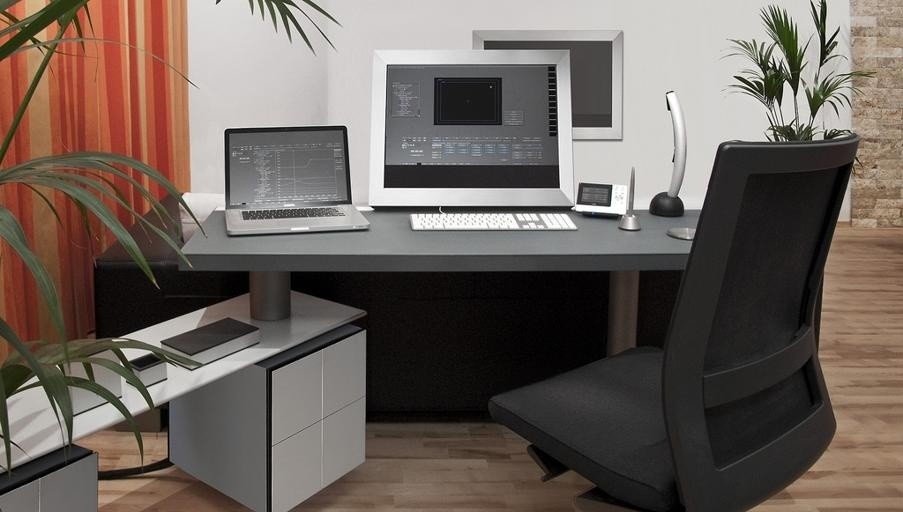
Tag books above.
[161,317,260,371]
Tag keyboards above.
[409,213,578,232]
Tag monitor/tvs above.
[368,49,576,208]
[471,29,624,142]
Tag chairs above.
[488,129,867,512]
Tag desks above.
[1,208,702,511]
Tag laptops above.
[223,125,371,235]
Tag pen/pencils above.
[628,167,635,217]
